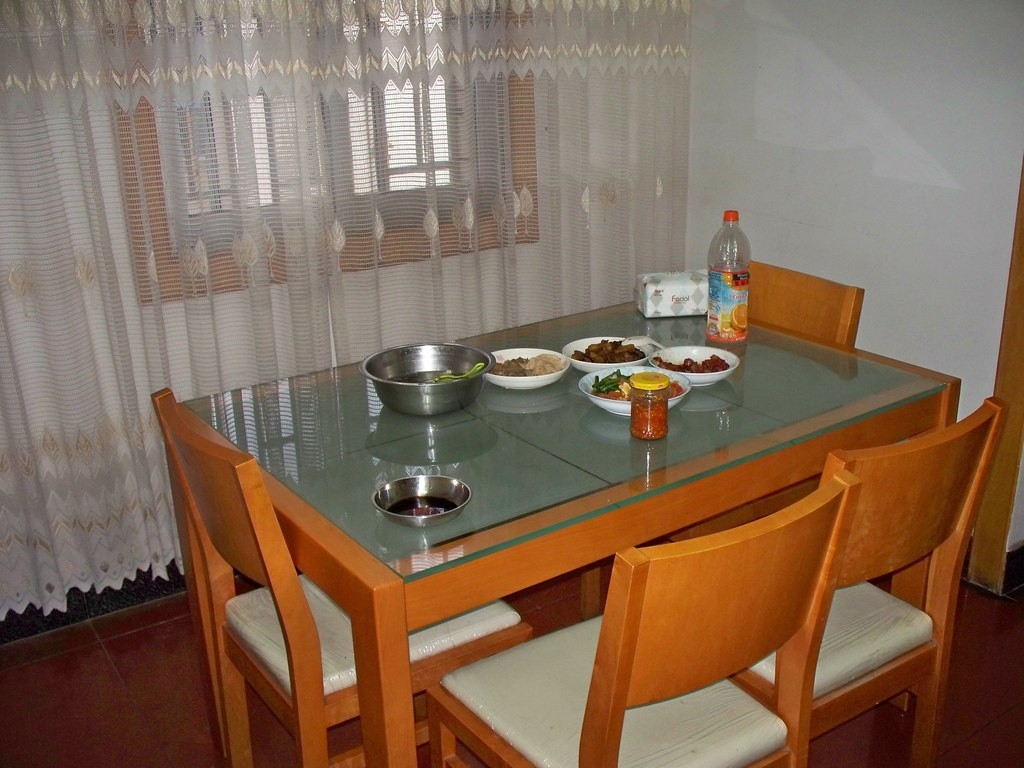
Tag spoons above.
[621,336,666,350]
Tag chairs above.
[426,468,862,768]
[722,393,1013,768]
[664,261,865,544]
[151,389,530,768]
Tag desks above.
[172,298,963,768]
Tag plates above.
[578,366,693,417]
[649,346,741,387]
[482,348,571,389]
[561,336,654,373]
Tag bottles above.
[628,372,670,440]
[704,210,751,344]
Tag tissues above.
[645,316,707,346]
[633,268,708,319]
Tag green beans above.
[592,369,632,394]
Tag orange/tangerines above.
[731,302,747,330]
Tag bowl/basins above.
[358,342,496,417]
[371,475,472,528]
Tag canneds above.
[629,372,669,440]
